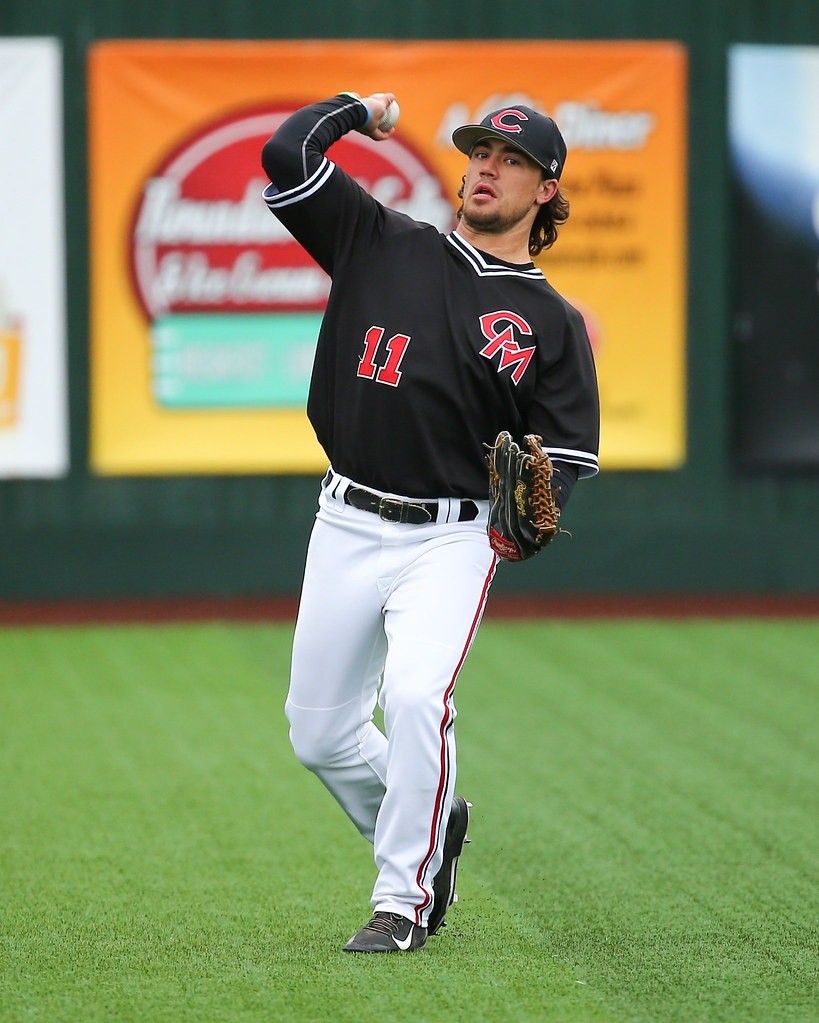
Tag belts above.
[323,471,478,525]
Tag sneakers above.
[344,908,429,954]
[429,796,472,933]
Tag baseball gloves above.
[484,430,560,562]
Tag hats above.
[452,104,567,182]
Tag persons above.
[261,91,600,952]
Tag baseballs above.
[368,93,400,133]
